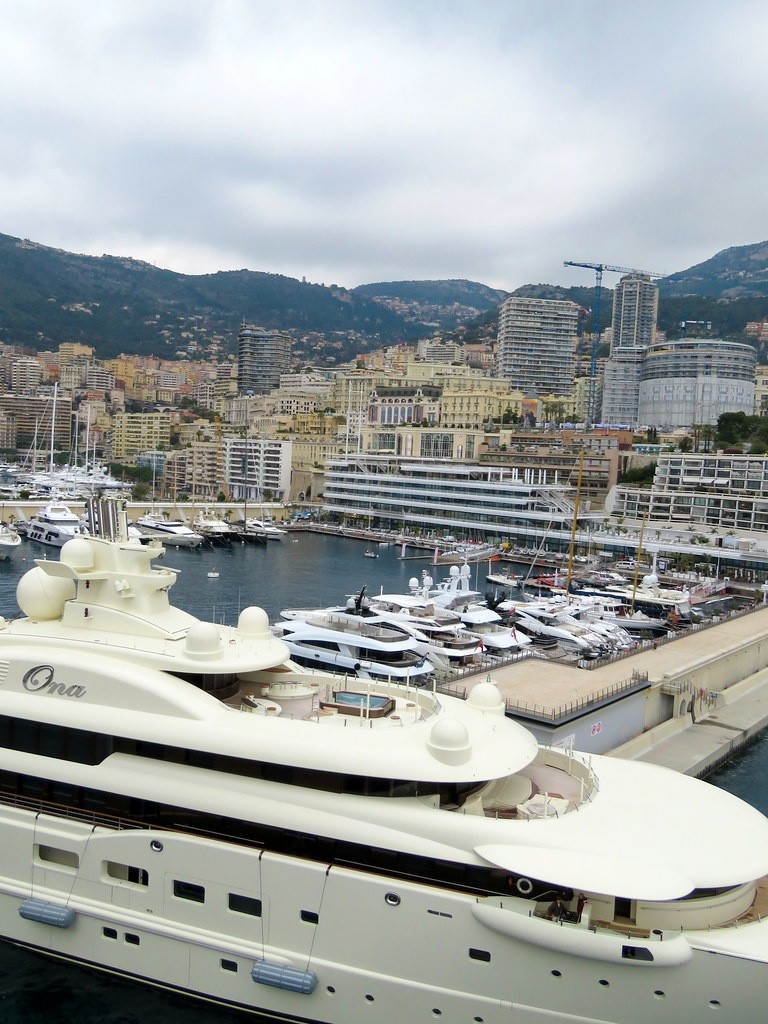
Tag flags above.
[506,607,515,617]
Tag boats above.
[0,495,768,1024]
[136,504,204,549]
[275,568,728,683]
[183,502,288,541]
[10,487,91,549]
[364,550,377,559]
[0,520,21,559]
[79,497,142,544]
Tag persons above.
[462,605,469,613]
[576,892,587,923]
[547,895,570,921]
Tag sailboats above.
[516,444,669,628]
